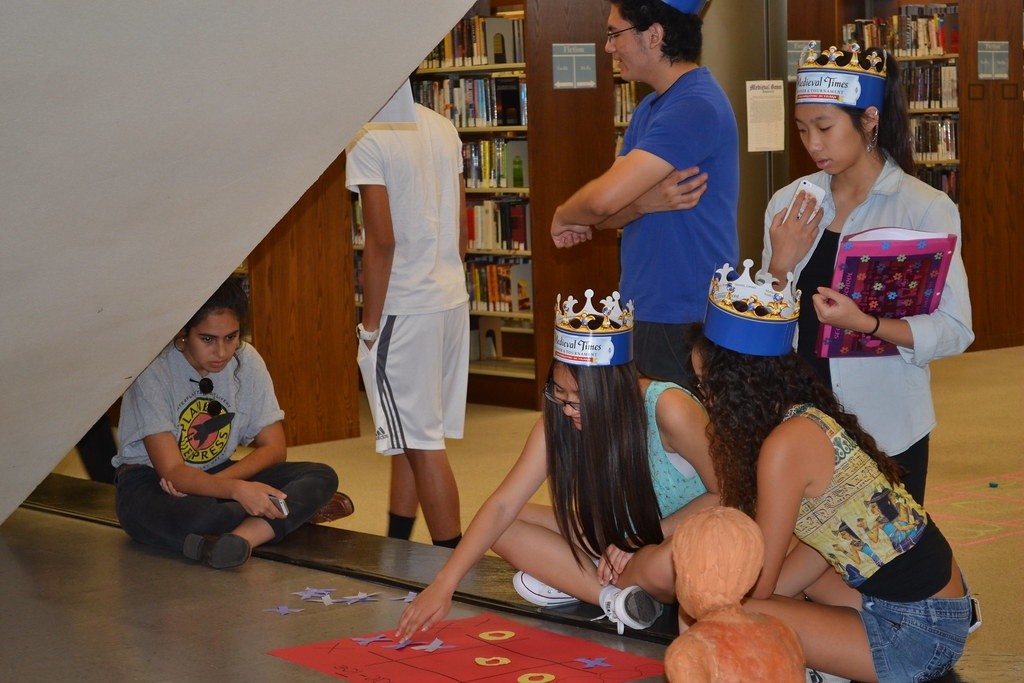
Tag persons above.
[345,69,470,548]
[110,280,355,570]
[396,289,724,644]
[678,259,970,683]
[762,41,974,506]
[664,506,806,683]
[550,0,739,396]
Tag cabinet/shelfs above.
[353,1,621,411]
[612,0,772,282]
[234,150,361,447]
[787,0,1024,351]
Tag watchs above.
[356,323,379,340]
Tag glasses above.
[544,371,584,415]
[606,24,638,39]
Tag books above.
[612,52,653,236]
[841,3,959,208]
[352,15,533,360]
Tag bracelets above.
[866,314,880,335]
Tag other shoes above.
[590,587,663,636]
[512,571,581,607]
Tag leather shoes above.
[182,530,253,571]
[306,492,356,522]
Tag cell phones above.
[268,496,290,515]
[783,180,825,225]
